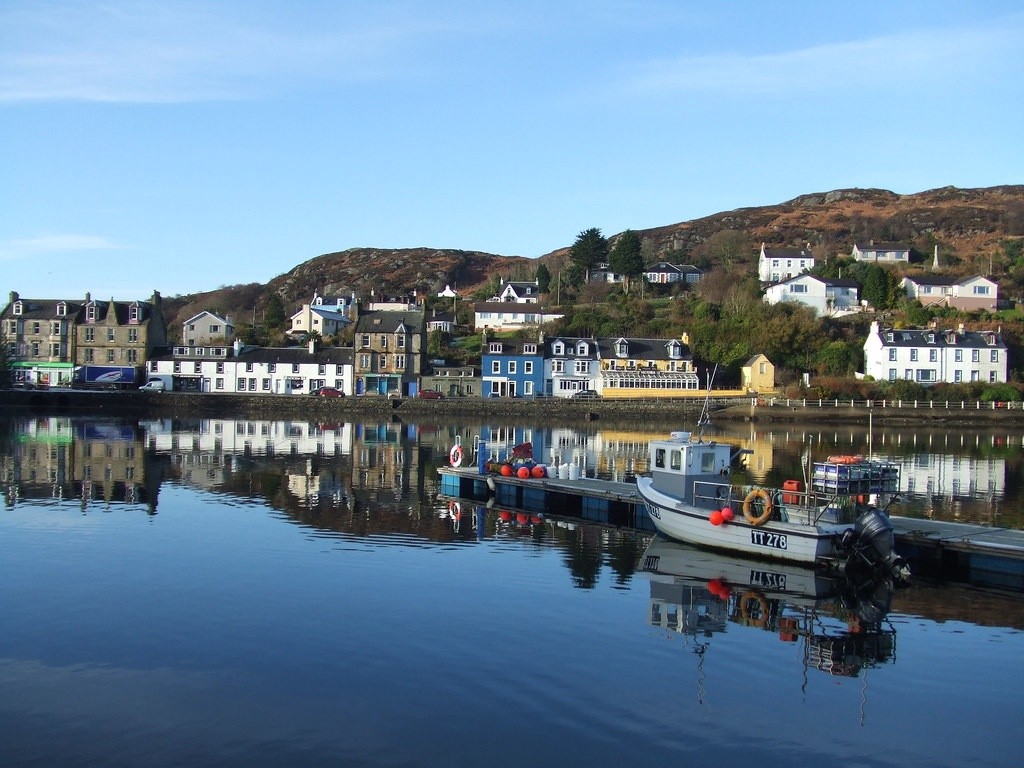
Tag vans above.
[139,381,166,394]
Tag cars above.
[417,389,444,400]
[321,388,345,398]
[573,390,598,398]
[308,386,326,396]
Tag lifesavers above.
[743,488,773,526]
[449,501,461,520]
[446,443,465,470]
[740,590,770,627]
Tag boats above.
[635,530,903,684]
[812,452,905,487]
[635,430,914,587]
